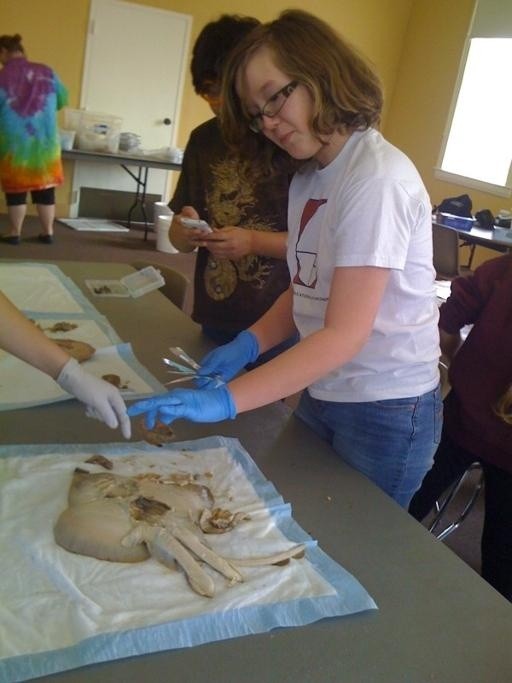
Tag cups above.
[60,130,76,150]
[154,201,174,231]
[156,215,180,254]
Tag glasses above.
[245,79,299,134]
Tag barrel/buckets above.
[153,202,178,233]
[156,215,184,254]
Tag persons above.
[0,291,132,442]
[0,34,69,245]
[408,254,511,605]
[166,15,301,372]
[125,9,444,514]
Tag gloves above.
[195,328,260,390]
[126,386,239,429]
[55,352,133,443]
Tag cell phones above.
[183,220,213,234]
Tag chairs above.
[432,222,477,283]
[130,259,190,312]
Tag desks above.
[55,145,185,242]
[2,259,512,683]
[433,212,512,270]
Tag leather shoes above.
[0,233,20,245]
[38,232,55,243]
